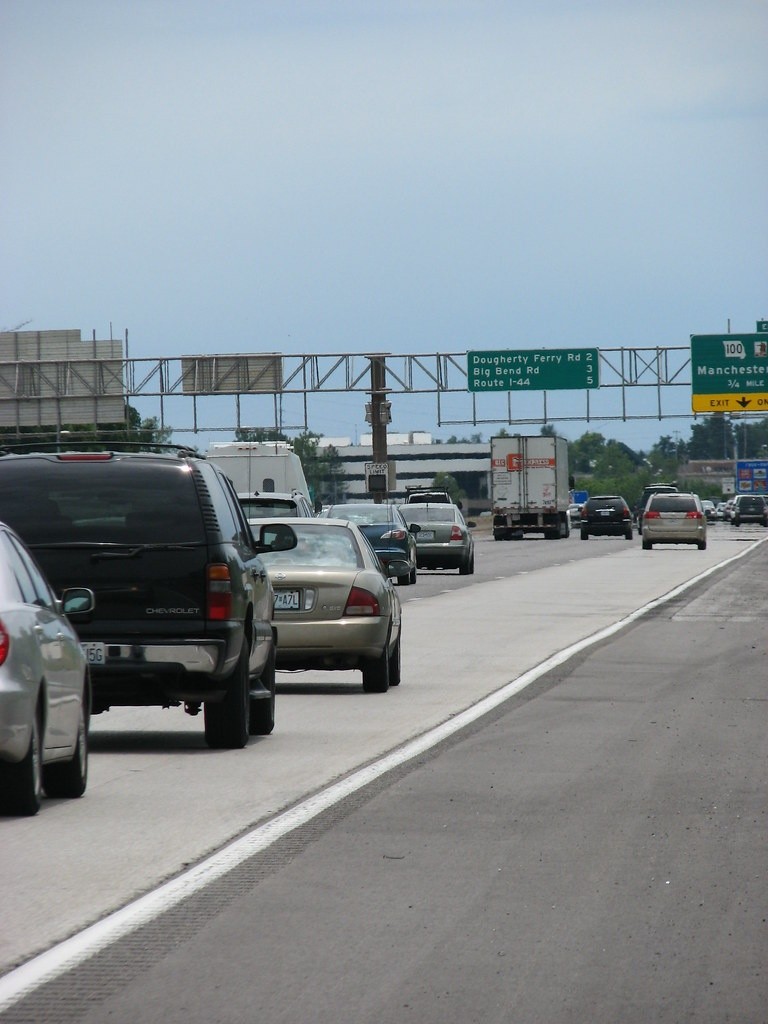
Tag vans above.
[567,490,589,524]
[636,482,679,535]
[201,440,322,549]
[404,485,463,510]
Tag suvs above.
[0,439,298,750]
[640,491,712,550]
[578,495,635,540]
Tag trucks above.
[490,436,574,541]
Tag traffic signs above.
[690,333,768,412]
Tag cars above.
[700,500,717,520]
[239,515,411,693]
[393,501,477,575]
[733,494,768,528]
[715,502,728,521]
[0,520,96,816]
[317,503,421,586]
[721,498,736,522]
